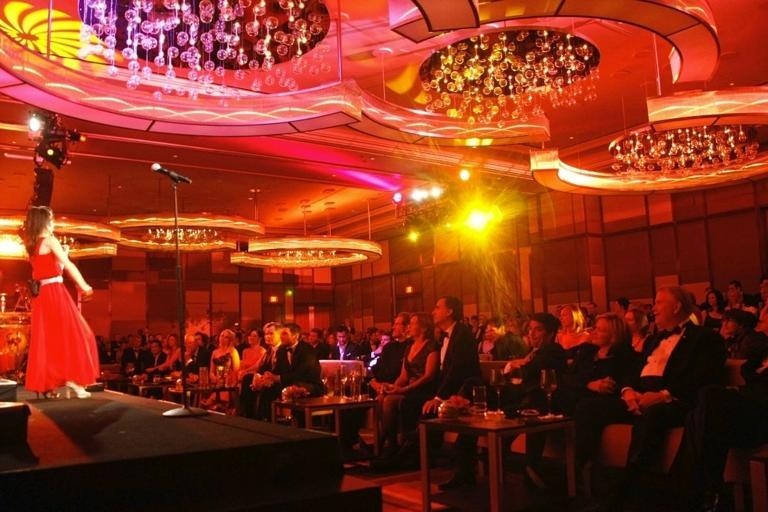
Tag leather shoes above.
[439,477,472,492]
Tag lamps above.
[1,1,363,137]
[348,1,718,146]
[529,35,767,195]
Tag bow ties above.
[440,331,449,338]
[661,326,681,339]
[285,347,293,354]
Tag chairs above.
[478,360,516,391]
[314,360,363,396]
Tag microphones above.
[150,162,193,184]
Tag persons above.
[20,205,101,399]
[96,279,768,512]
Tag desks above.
[278,397,381,461]
[419,415,579,511]
[127,380,163,398]
[168,383,234,417]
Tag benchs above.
[417,422,745,482]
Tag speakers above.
[29,167,55,206]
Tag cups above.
[472,385,488,415]
[153,375,160,384]
[199,367,208,388]
[216,365,225,386]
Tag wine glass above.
[539,370,558,416]
[320,367,327,399]
[337,365,347,398]
[490,369,505,414]
[349,364,361,400]
[509,364,522,415]
[364,366,373,400]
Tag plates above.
[164,376,171,380]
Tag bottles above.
[226,367,233,387]
[177,377,183,391]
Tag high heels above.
[37,389,62,400]
[65,381,91,399]
